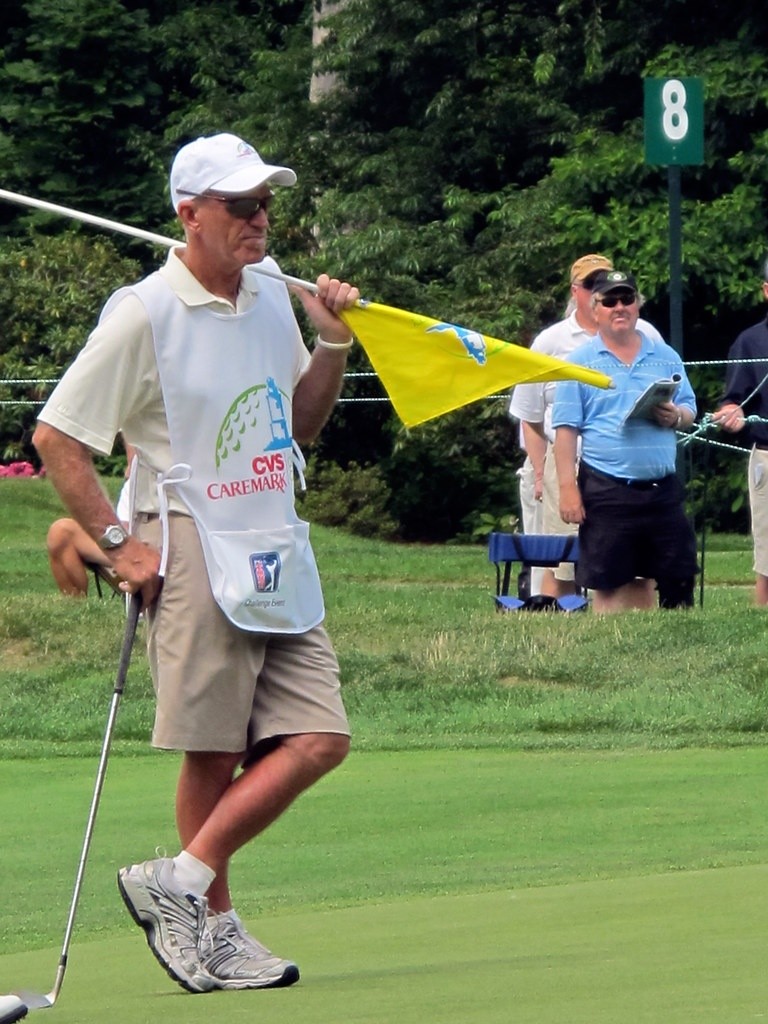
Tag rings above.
[539,497,543,502]
[665,417,667,422]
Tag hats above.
[170,133,297,216]
[571,254,614,283]
[592,270,635,294]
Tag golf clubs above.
[0,584,145,1024]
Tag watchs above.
[96,523,131,552]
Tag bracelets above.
[673,407,681,429]
[316,333,354,349]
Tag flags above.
[332,296,616,430]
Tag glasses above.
[595,295,636,307]
[176,189,275,219]
[574,276,594,291]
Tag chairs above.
[488,532,589,612]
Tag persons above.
[710,258,768,611]
[506,255,696,614]
[46,451,132,605]
[29,131,361,996]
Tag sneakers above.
[117,846,300,993]
